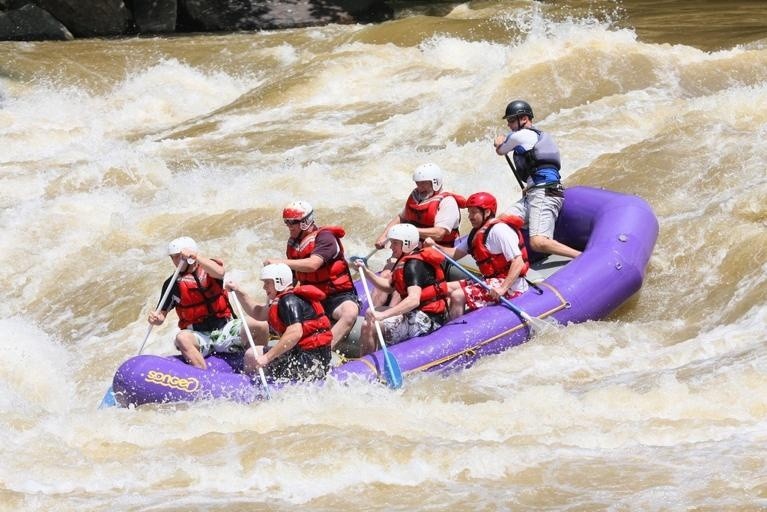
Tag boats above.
[110,180,664,408]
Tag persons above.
[146,234,269,371]
[260,201,361,351]
[369,161,468,310]
[352,222,449,359]
[492,97,582,266]
[423,189,531,324]
[222,261,333,380]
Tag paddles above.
[99,258,185,416]
[352,244,382,267]
[230,289,272,400]
[356,266,403,388]
[431,245,560,345]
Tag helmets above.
[466,192,497,218]
[167,236,198,265]
[282,201,314,231]
[259,263,294,292]
[414,164,443,191]
[503,101,533,119]
[387,223,420,254]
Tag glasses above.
[506,115,524,123]
[284,209,314,226]
